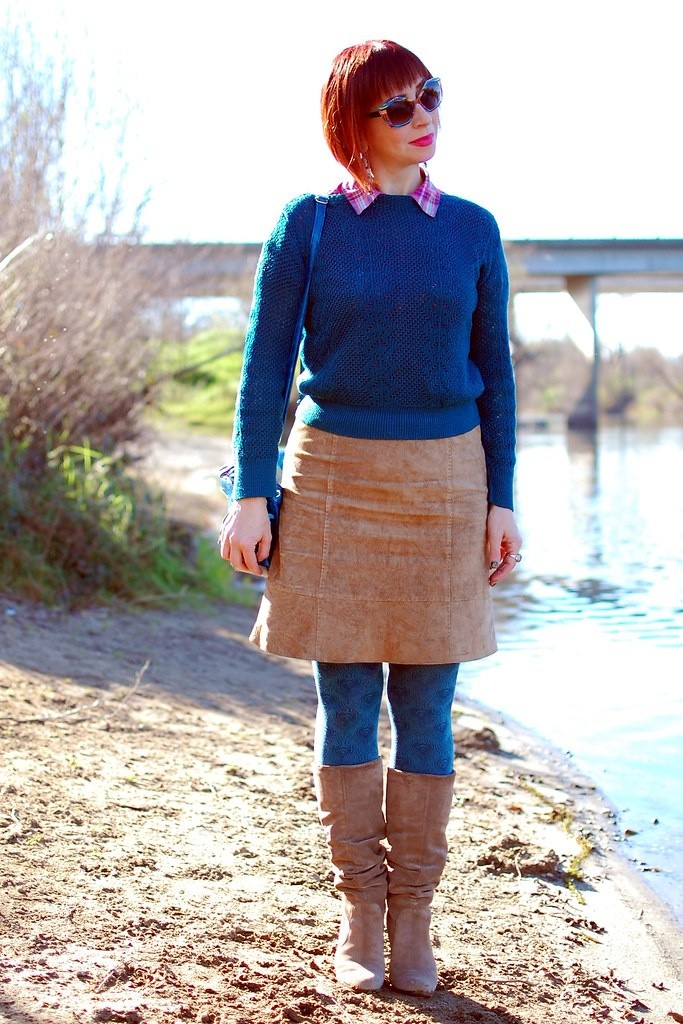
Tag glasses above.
[369,77,444,128]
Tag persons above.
[219,38,520,996]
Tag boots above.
[385,767,456,997]
[312,755,387,993]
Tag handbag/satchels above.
[217,450,282,578]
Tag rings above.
[509,553,522,562]
